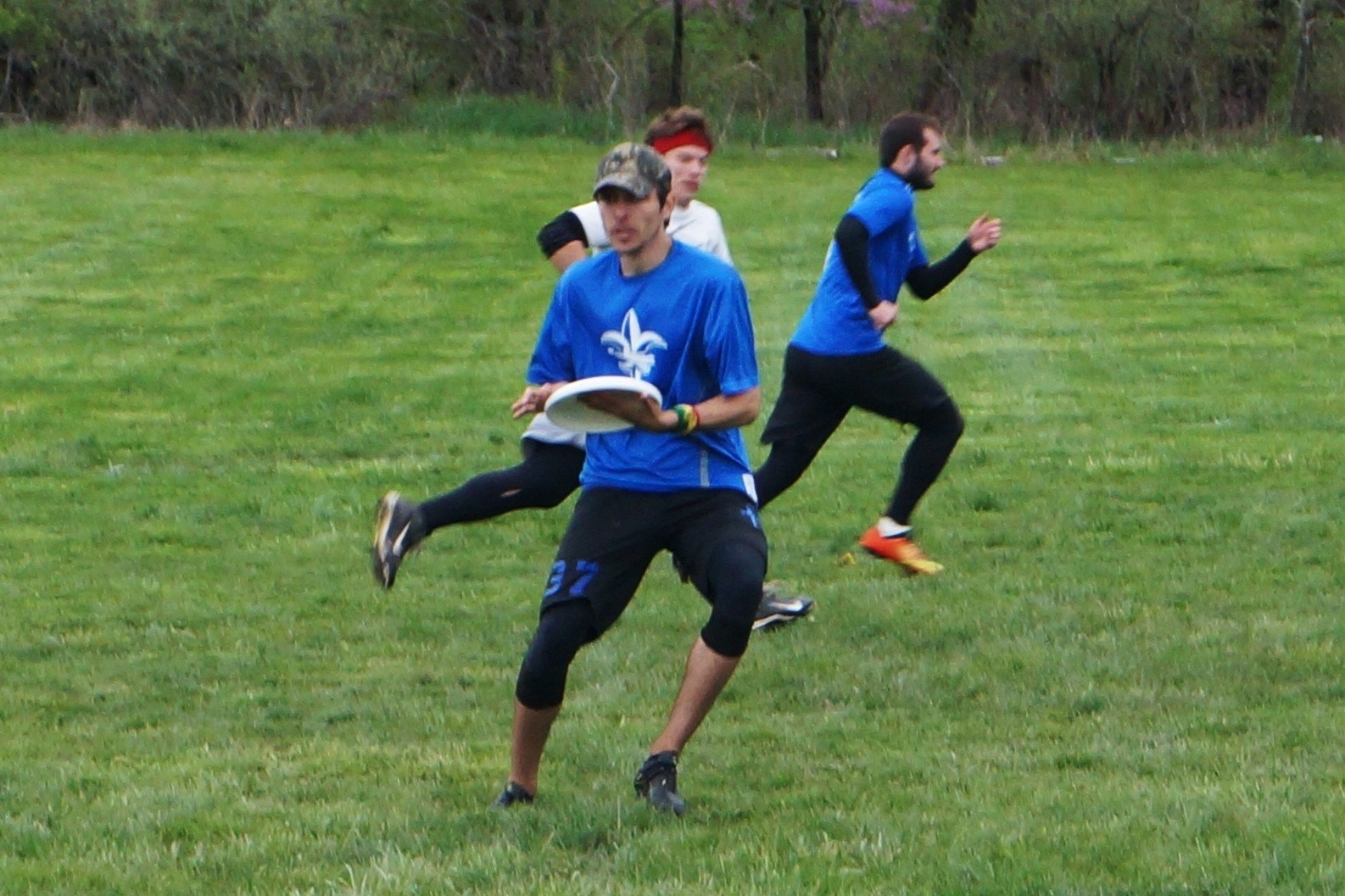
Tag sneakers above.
[496,781,534,809]
[751,580,815,630]
[860,525,944,575]
[634,754,685,814]
[370,489,425,591]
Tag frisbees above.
[543,374,663,433]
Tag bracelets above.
[671,404,700,437]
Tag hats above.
[592,141,671,199]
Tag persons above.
[370,105,814,633]
[753,112,1003,574]
[493,142,767,817]
